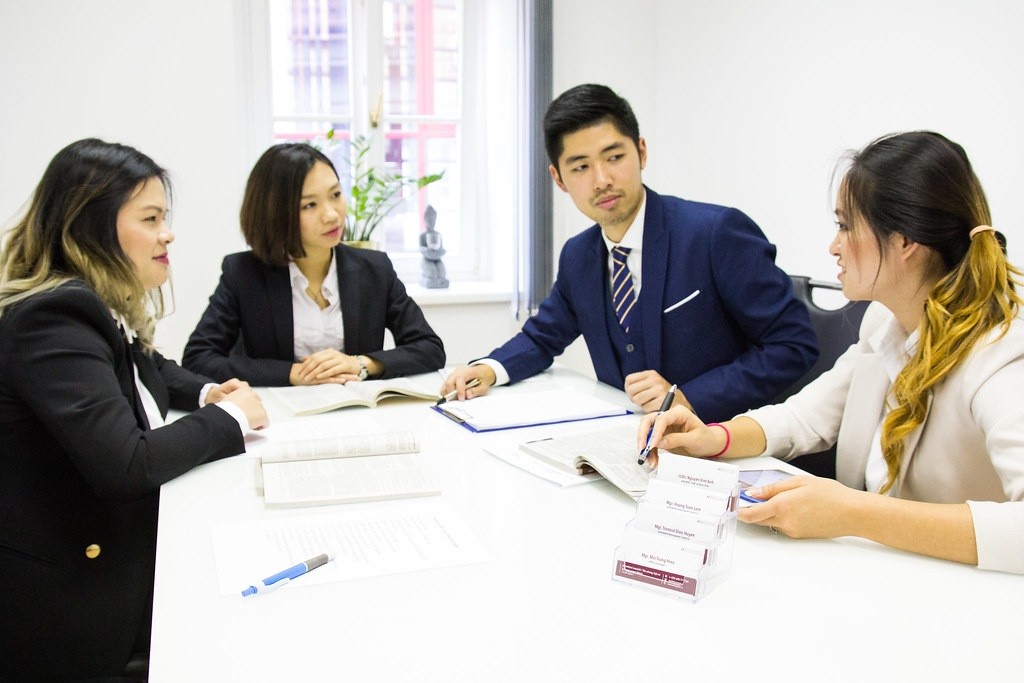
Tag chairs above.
[759,277,871,479]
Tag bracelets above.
[707,423,731,461]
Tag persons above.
[439,83,821,424]
[0,137,269,683]
[181,142,446,387]
[636,129,1024,576]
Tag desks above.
[147,360,1024,683]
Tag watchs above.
[355,355,369,381]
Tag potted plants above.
[307,127,445,249]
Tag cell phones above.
[739,469,799,505]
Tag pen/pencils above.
[434,377,482,406]
[241,550,336,598]
[636,384,678,466]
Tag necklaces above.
[307,287,321,305]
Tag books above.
[519,423,726,505]
[268,377,453,419]
[261,430,441,511]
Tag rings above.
[770,525,778,535]
